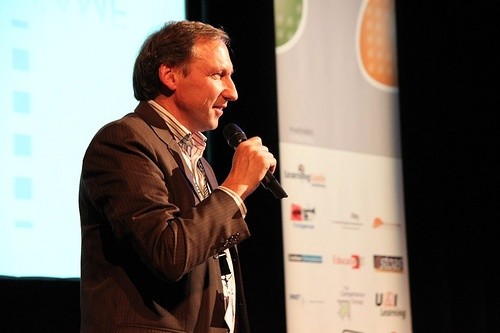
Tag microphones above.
[223,123,288,200]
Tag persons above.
[76,18,279,333]
